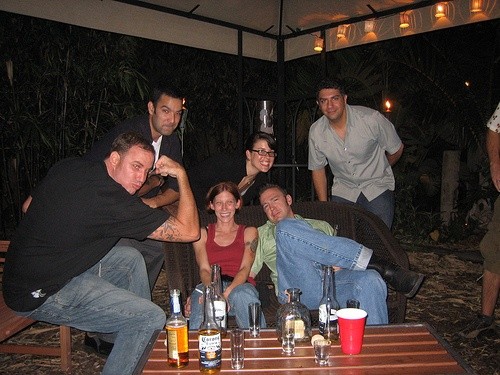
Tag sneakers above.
[459,314,495,339]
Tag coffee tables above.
[131,321,477,375]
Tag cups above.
[336,308,368,354]
[280,333,295,354]
[248,303,260,337]
[314,339,332,367]
[229,329,244,370]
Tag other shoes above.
[84,333,115,355]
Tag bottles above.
[197,286,222,375]
[318,266,340,343]
[276,288,313,344]
[210,264,228,338]
[164,289,189,368]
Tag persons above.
[458,103,500,339]
[2,75,425,375]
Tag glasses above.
[251,149,278,158]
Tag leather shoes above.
[386,263,424,298]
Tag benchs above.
[0,241,70,375]
[163,200,409,323]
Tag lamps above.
[435,3,445,17]
[246,88,276,135]
[379,87,394,118]
[399,14,409,28]
[470,0,482,12]
[314,38,323,50]
[364,21,374,33]
[337,25,346,37]
